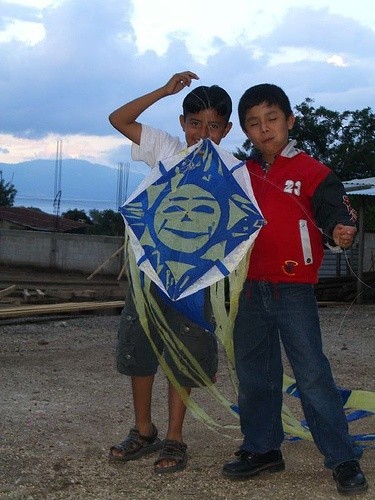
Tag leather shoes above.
[222,447,285,481]
[333,460,369,496]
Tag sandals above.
[154,440,188,474]
[108,423,161,462]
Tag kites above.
[118,138,375,456]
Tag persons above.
[109,71,233,473]
[222,83,368,496]
